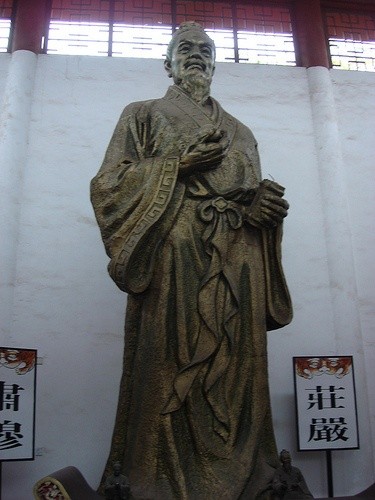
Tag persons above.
[272,446,317,500]
[89,23,289,500]
